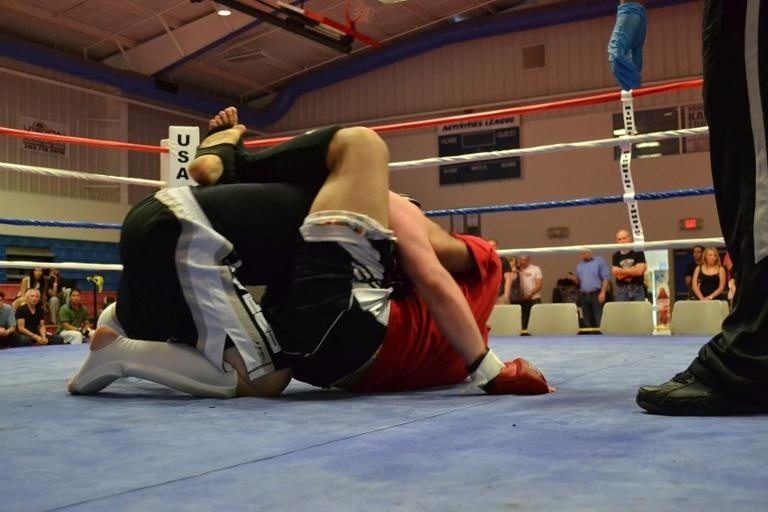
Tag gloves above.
[607,2,647,91]
[466,347,549,395]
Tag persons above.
[606,0,768,416]
[189,106,501,389]
[69,184,555,396]
[486,230,738,329]
[0,268,114,344]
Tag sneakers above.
[636,369,768,416]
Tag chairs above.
[525,302,580,335]
[668,299,730,337]
[486,303,523,335]
[598,300,656,336]
